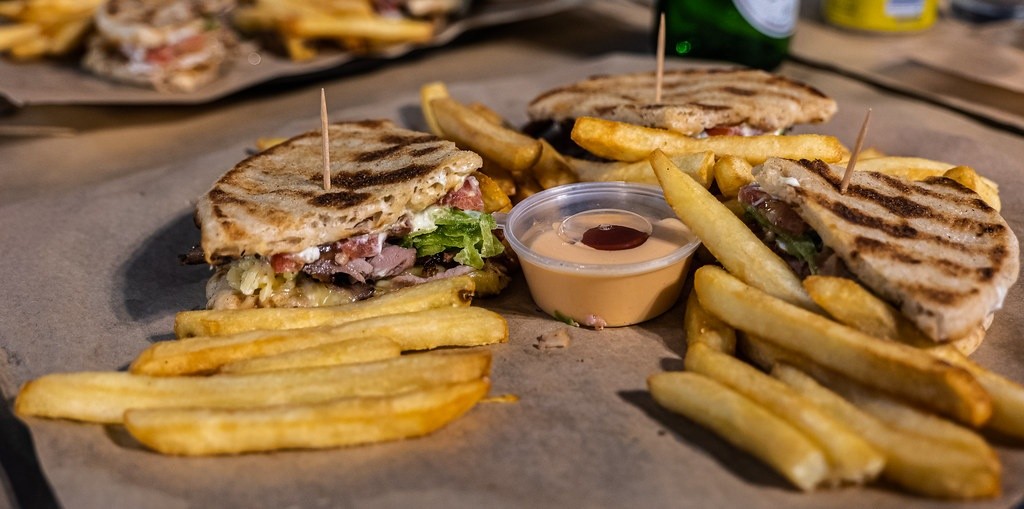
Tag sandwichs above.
[522,64,839,139]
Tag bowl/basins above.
[503,180,707,329]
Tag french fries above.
[11,80,1024,500]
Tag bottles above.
[648,0,803,74]
